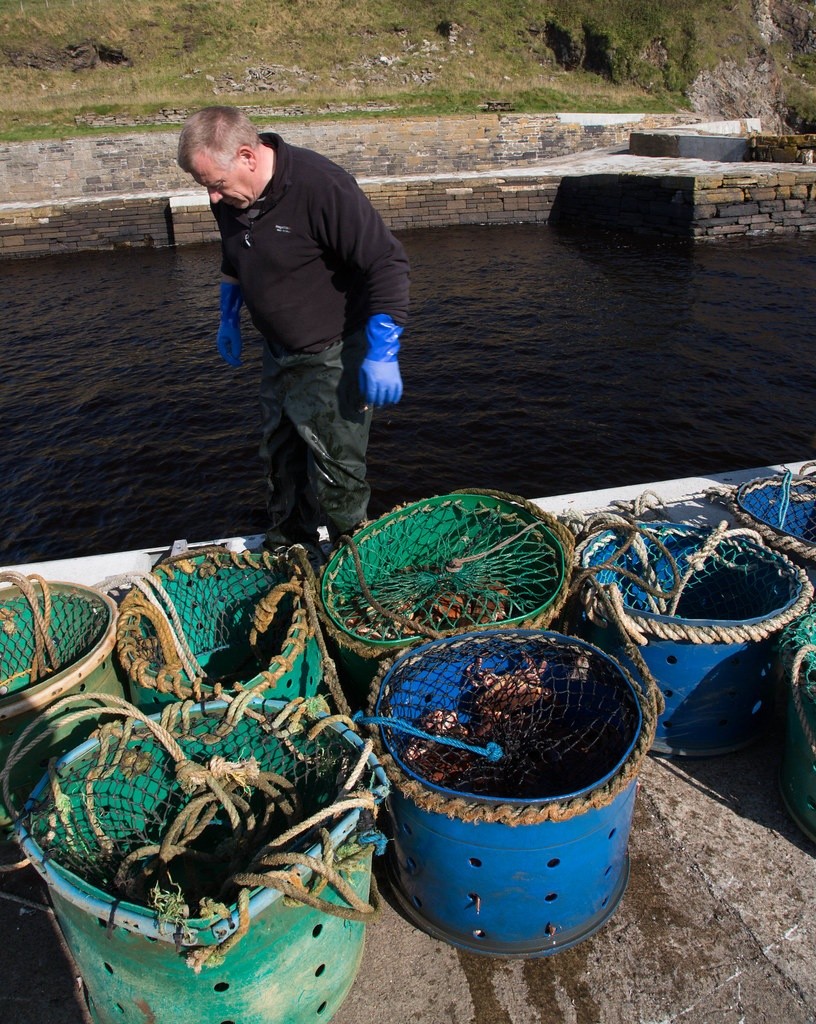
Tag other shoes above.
[243,542,276,555]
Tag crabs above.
[397,650,556,786]
[347,562,511,640]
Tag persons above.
[178,106,412,556]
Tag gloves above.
[358,313,403,407]
[216,281,243,368]
